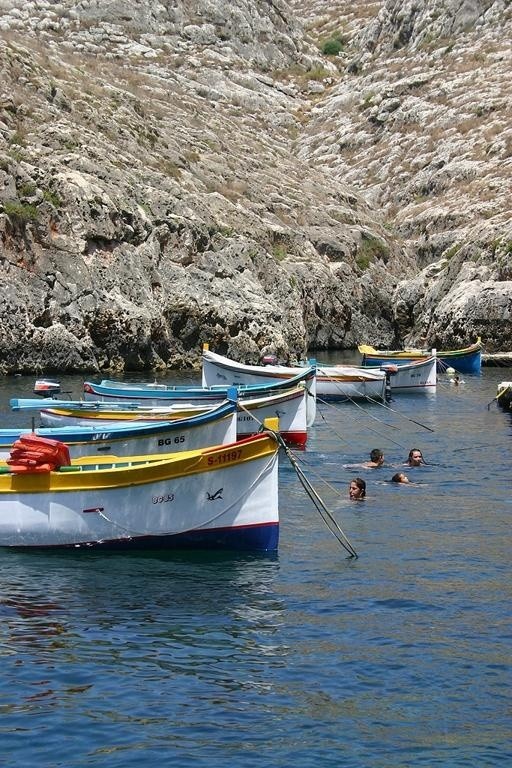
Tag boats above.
[291,348,437,394]
[82,359,318,430]
[0,417,282,554]
[38,380,309,451]
[357,336,484,377]
[497,381,512,412]
[200,342,398,404]
[0,387,239,473]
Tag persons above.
[342,448,400,470]
[347,477,366,501]
[402,448,438,466]
[378,472,423,488]
[453,376,460,386]
